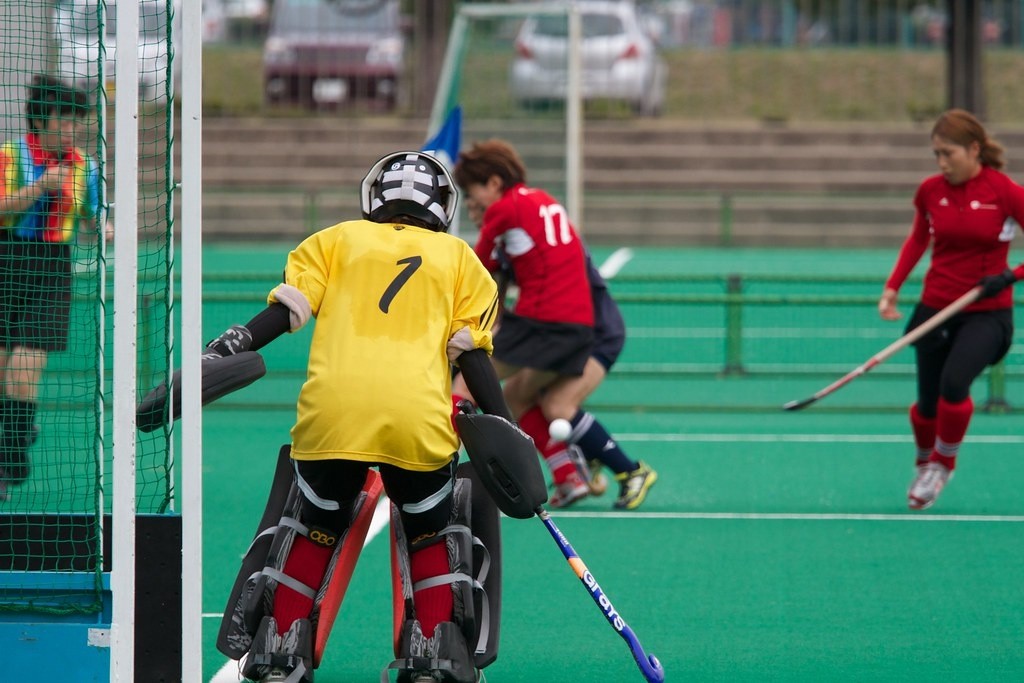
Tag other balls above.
[548,417,573,442]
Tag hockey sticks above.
[536,503,664,683]
[567,443,606,496]
[780,285,983,412]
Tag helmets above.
[373,155,450,228]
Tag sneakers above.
[584,455,607,495]
[616,459,659,510]
[549,473,588,507]
[908,462,955,509]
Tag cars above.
[48,0,183,105]
[510,0,669,118]
[260,0,420,114]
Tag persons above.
[0,74,115,500]
[447,140,659,510]
[876,111,1024,508]
[136,151,546,681]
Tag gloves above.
[199,324,252,360]
[972,268,1016,303]
